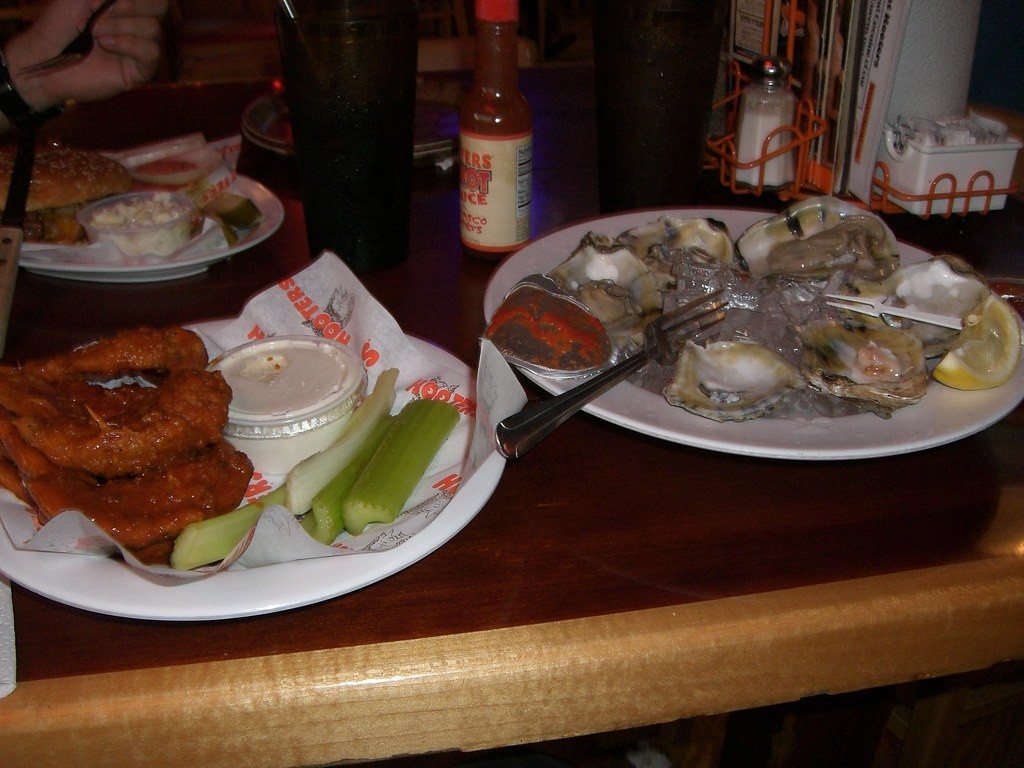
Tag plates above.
[484,205,1024,460]
[16,148,286,284]
[241,85,465,162]
[0,318,515,622]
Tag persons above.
[0,0,169,135]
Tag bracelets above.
[0,50,65,131]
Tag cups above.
[273,3,415,272]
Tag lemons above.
[931,291,1021,391]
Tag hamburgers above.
[1,143,132,245]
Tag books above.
[726,0,911,207]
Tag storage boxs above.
[873,127,1022,216]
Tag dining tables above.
[0,60,1024,767]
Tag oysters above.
[547,194,995,424]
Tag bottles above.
[456,0,533,260]
[733,56,794,191]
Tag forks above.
[494,285,742,461]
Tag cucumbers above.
[203,190,262,228]
